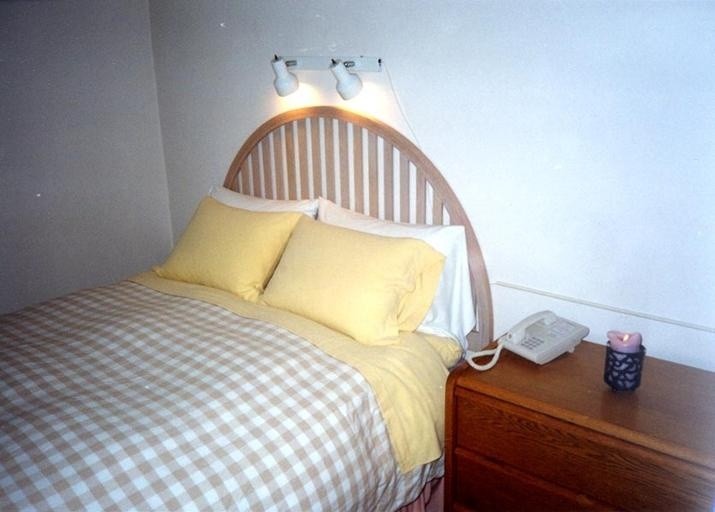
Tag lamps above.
[268,52,385,103]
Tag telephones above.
[497,311,589,365]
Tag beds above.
[0,104,495,511]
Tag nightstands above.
[442,327,714,512]
